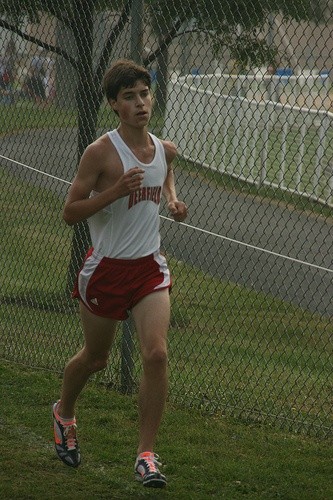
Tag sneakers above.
[134,452,168,488]
[53,400,81,468]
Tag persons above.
[51,58,190,489]
[0,51,57,111]
